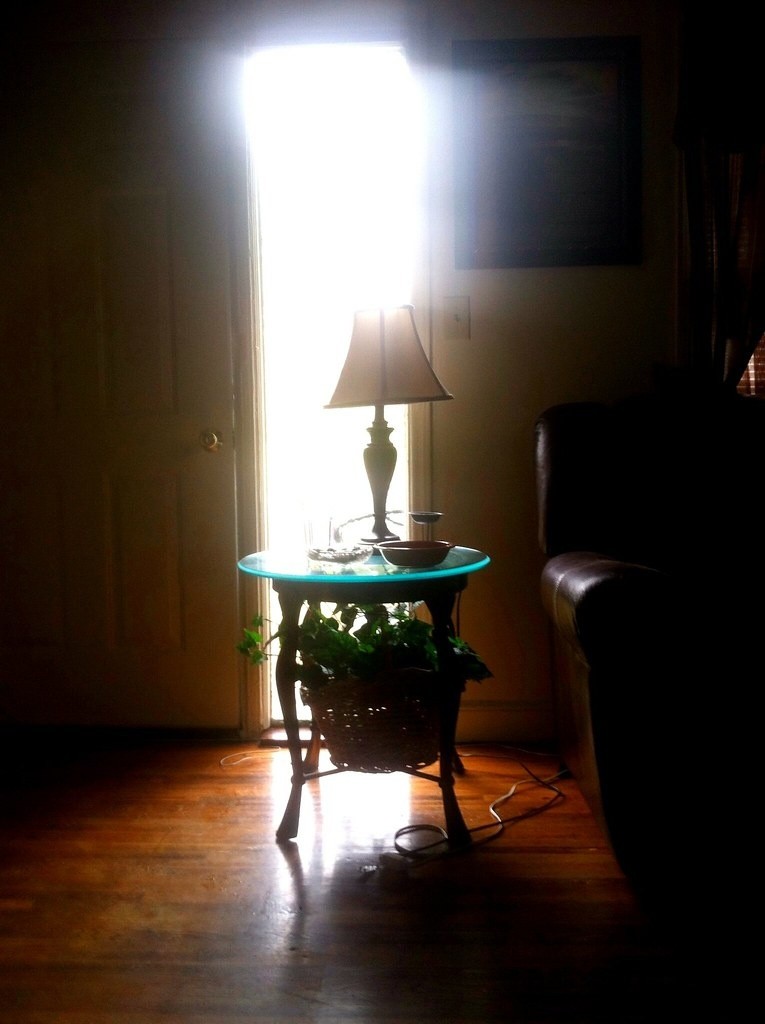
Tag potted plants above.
[239,601,493,773]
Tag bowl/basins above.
[309,547,373,563]
[409,512,444,524]
[372,540,456,569]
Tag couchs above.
[532,397,763,916]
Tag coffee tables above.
[237,544,491,848]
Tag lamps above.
[323,303,454,555]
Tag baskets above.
[302,678,439,773]
